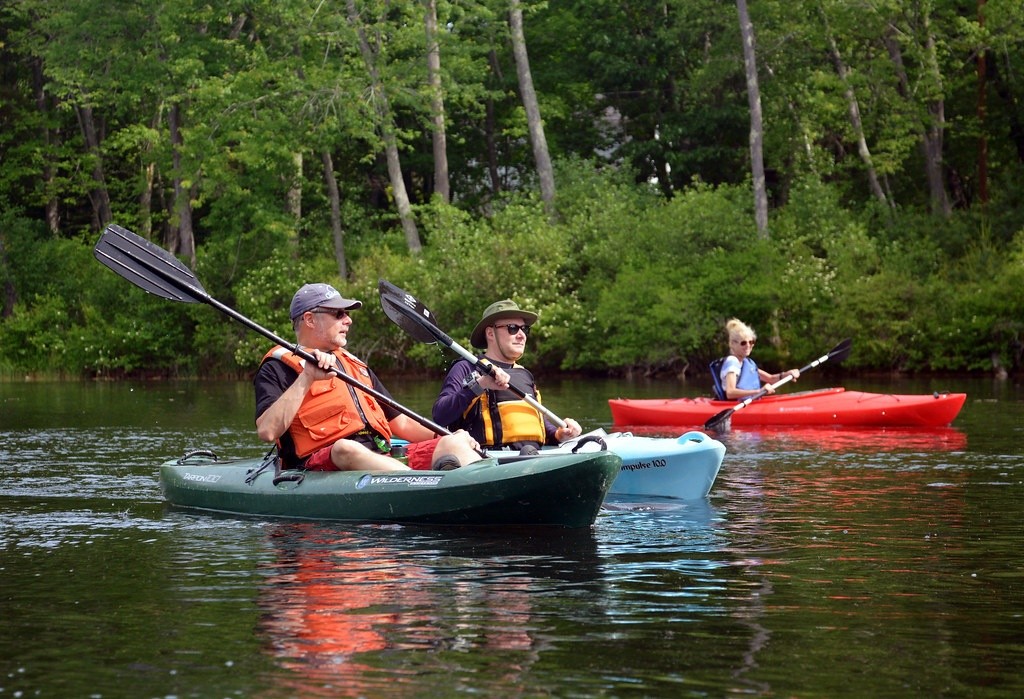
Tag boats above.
[158,450,622,535]
[609,387,967,427]
[383,427,727,501]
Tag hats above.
[470,300,538,349]
[290,283,362,320]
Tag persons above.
[431,299,582,451]
[720,318,800,401]
[253,283,482,470]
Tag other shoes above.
[433,454,461,470]
[519,445,539,455]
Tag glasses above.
[313,308,349,319]
[733,340,753,345]
[495,324,530,336]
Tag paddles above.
[90,220,492,459]
[702,334,855,432]
[377,275,570,433]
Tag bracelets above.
[433,433,440,439]
[779,372,782,380]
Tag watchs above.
[463,371,484,396]
